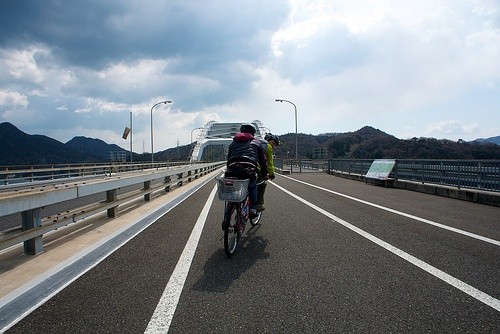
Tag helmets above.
[239,124,257,133]
[265,133,280,145]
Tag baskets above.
[214,176,250,201]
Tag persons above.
[221,124,269,230]
[257,132,279,213]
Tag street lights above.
[275,99,301,173]
[191,127,203,156]
[151,101,172,163]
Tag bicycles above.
[215,173,274,258]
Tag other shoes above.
[258,204,265,211]
[250,209,260,218]
